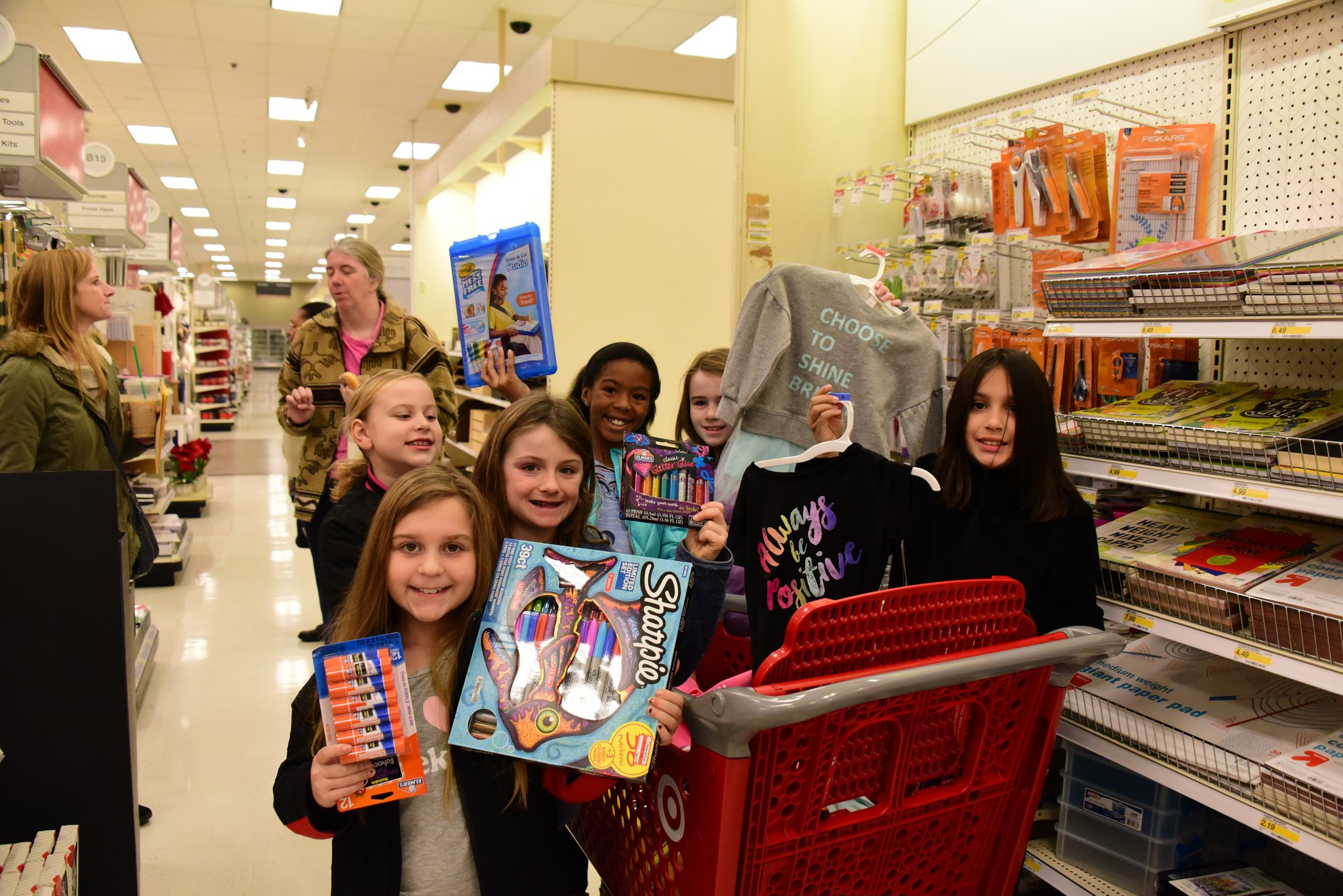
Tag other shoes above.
[298,623,326,641]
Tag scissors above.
[1071,339,1089,403]
[1099,394,1126,406]
[1047,343,1059,397]
[1112,350,1126,382]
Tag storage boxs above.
[1055,738,1184,896]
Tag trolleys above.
[564,574,1128,896]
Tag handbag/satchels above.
[128,489,159,580]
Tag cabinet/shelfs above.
[108,290,254,587]
[1023,262,1343,896]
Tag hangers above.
[753,399,941,492]
[849,248,905,316]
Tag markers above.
[514,598,622,704]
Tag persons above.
[0,248,141,569]
[488,273,533,357]
[807,345,1103,634]
[462,305,473,319]
[272,464,685,896]
[278,238,751,687]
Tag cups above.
[124,377,159,438]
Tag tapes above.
[904,267,989,294]
[918,192,991,220]
